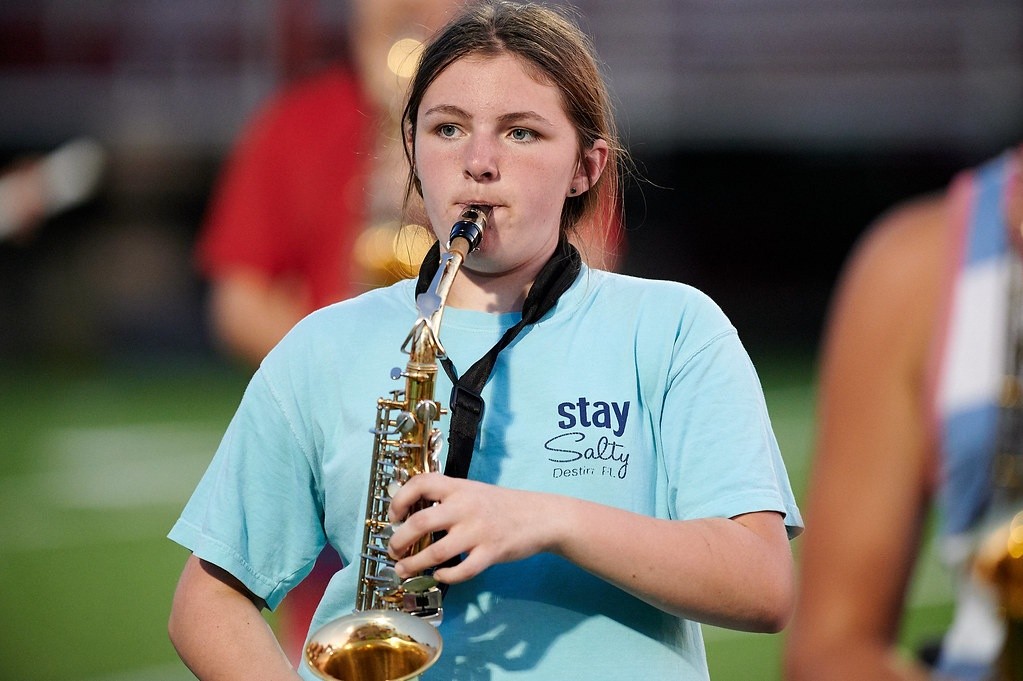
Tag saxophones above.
[300,200,502,681]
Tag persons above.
[165,1,805,681]
[194,0,625,660]
[783,151,1023,681]
